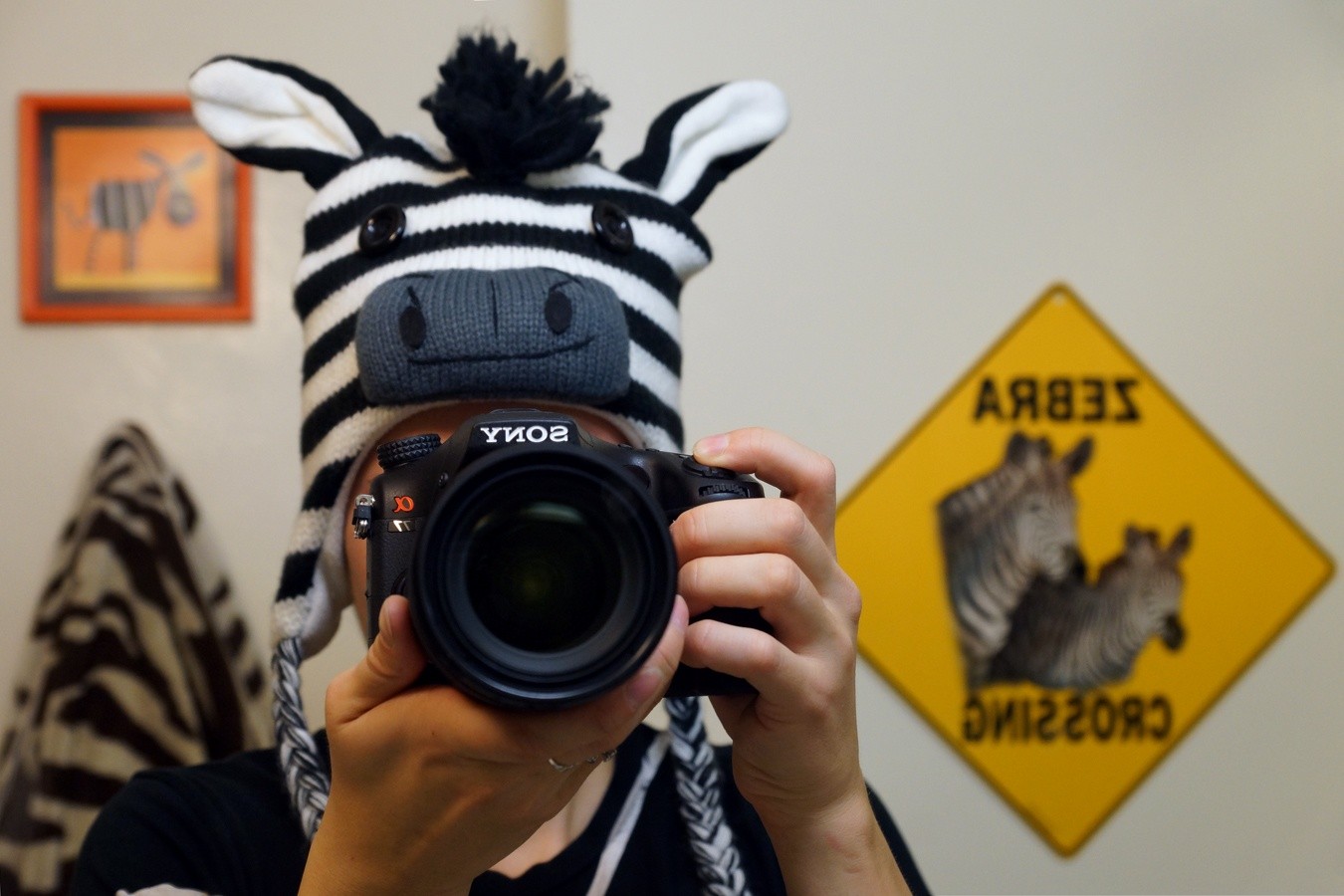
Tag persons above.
[29,34,936,896]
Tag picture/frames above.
[13,85,262,330]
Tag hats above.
[184,24,796,896]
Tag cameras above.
[349,409,769,715]
